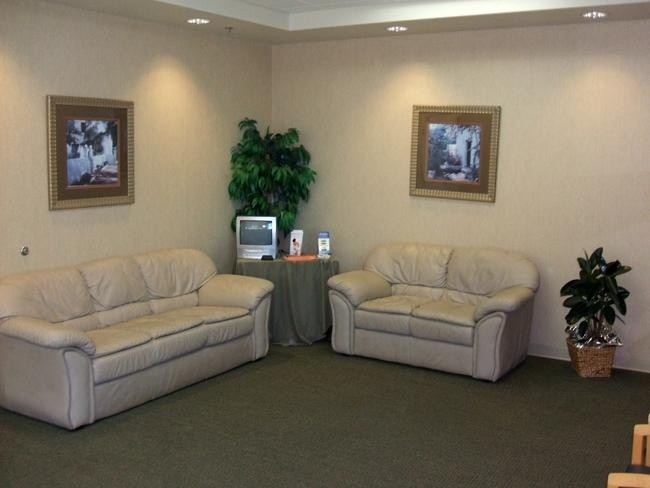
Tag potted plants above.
[558,244,634,380]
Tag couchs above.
[326,241,542,385]
[0,246,276,431]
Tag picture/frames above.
[45,94,136,213]
[409,104,502,203]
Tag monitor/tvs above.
[236,216,277,259]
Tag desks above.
[235,249,339,347]
